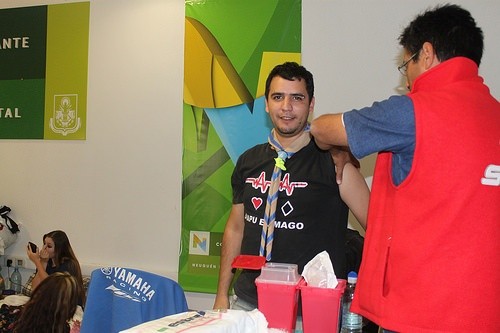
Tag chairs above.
[79,267,189,333]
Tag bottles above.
[340,277,362,333]
[10,268,21,293]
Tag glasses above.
[398,49,422,76]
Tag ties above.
[258,121,312,261]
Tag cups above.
[254,263,304,333]
[296,276,346,333]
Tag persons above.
[309,4,500,333]
[0,229,85,333]
[212,61,371,311]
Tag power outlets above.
[4,256,16,267]
